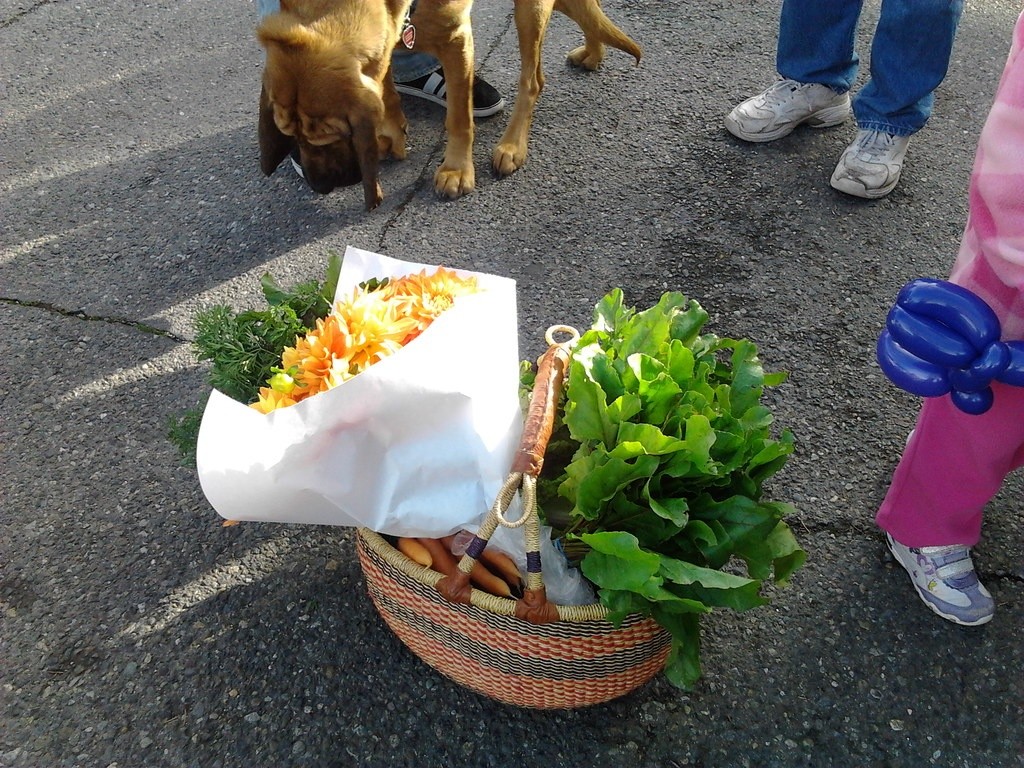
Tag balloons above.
[877,278,1024,415]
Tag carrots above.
[397,529,523,602]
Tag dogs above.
[258,0,641,206]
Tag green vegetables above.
[519,288,806,688]
[166,252,343,454]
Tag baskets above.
[356,343,675,708]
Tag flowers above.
[223,263,586,604]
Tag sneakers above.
[393,67,504,117]
[723,73,851,142]
[830,128,911,198]
[885,530,994,626]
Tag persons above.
[259,0,505,178]
[873,9,1024,626]
[724,0,967,199]
